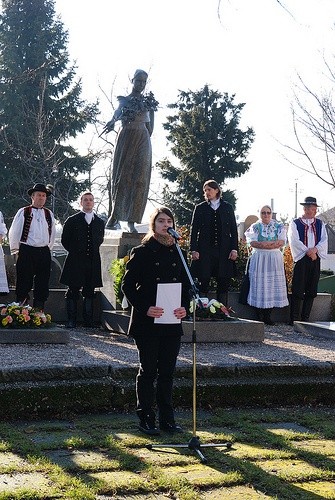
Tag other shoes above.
[263,319,275,326]
[138,420,159,434]
[159,420,181,432]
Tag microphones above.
[166,227,181,240]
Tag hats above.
[27,183,51,197]
[300,197,320,208]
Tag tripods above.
[145,241,231,463]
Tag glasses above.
[261,211,272,215]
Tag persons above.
[0,211,9,304]
[243,205,290,322]
[7,183,56,313]
[97,69,155,233]
[120,206,192,436]
[59,192,105,328]
[287,196,328,326]
[190,180,238,319]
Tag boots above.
[16,294,30,302]
[290,297,301,325]
[216,291,228,307]
[83,297,99,327]
[32,297,45,309]
[200,292,208,297]
[66,299,78,328]
[302,297,314,321]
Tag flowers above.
[0,303,51,329]
[189,296,233,321]
